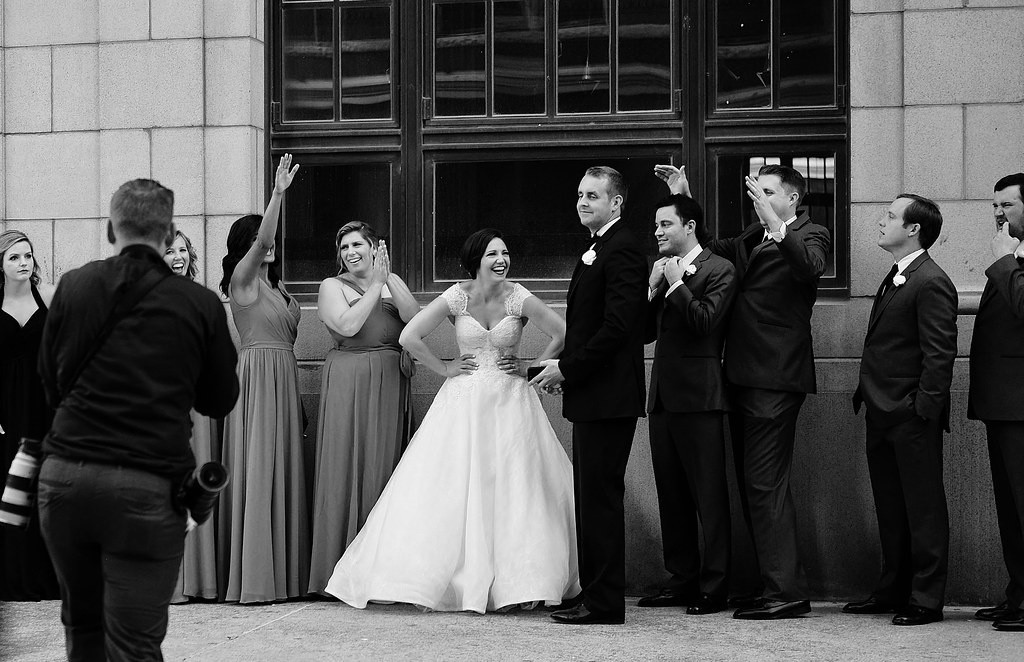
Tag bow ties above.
[584,234,600,251]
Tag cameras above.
[184,461,230,525]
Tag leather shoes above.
[637,589,690,606]
[892,604,944,625]
[992,610,1024,631]
[551,603,603,624]
[733,596,812,619]
[843,591,897,613]
[975,603,1012,621]
[686,592,737,614]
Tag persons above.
[0,229,56,602]
[307,221,420,606]
[526,166,647,624]
[162,228,219,606]
[36,178,240,662]
[967,172,1024,631]
[324,229,582,614]
[217,153,310,603]
[637,194,735,614]
[855,193,959,626]
[654,164,828,619]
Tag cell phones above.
[526,366,546,383]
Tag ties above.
[881,264,898,300]
[763,235,768,242]
